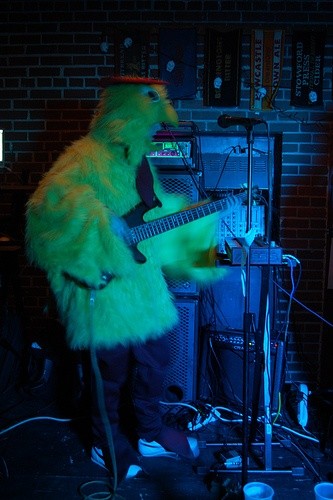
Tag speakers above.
[158,168,204,298]
[157,298,201,419]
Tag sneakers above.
[86,460,142,480]
[289,384,308,425]
[138,429,198,458]
[187,404,219,431]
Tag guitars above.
[63,183,261,290]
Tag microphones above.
[217,114,264,128]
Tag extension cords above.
[187,410,221,431]
[296,384,309,426]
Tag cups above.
[313,482,333,500]
[243,481,274,500]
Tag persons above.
[23,76,243,483]
